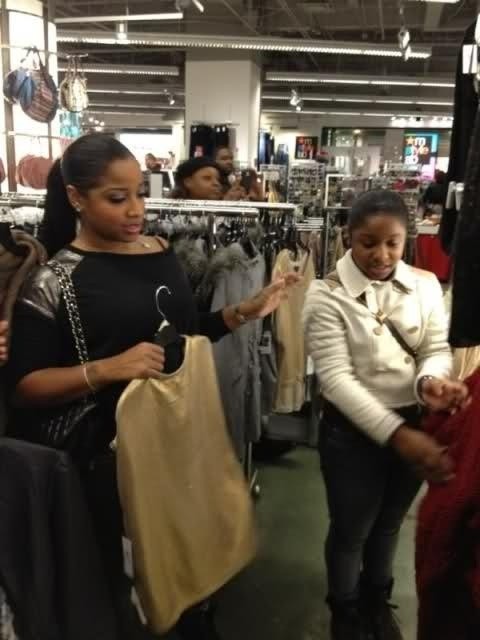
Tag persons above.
[0,321,11,367]
[423,172,445,221]
[301,189,472,640]
[141,132,288,200]
[7,131,301,640]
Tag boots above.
[324,573,403,640]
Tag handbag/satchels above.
[4,48,90,123]
[35,400,115,470]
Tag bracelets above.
[235,302,246,324]
[83,362,96,392]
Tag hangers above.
[129,284,186,374]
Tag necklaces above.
[139,237,152,249]
